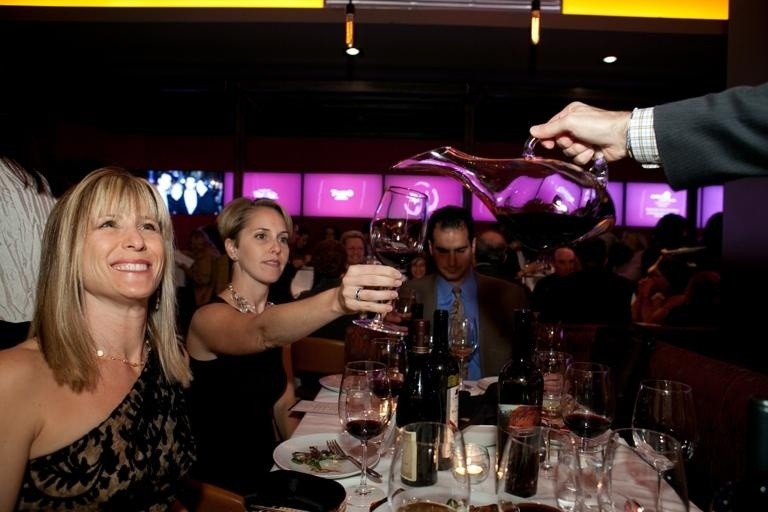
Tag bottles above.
[706,394,768,510]
[395,318,444,487]
[495,309,545,498]
[393,301,544,498]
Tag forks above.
[325,438,383,481]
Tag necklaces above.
[88,333,154,372]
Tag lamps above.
[529,1,542,45]
[342,0,362,57]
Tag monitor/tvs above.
[144,166,225,217]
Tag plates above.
[319,373,370,395]
[272,432,381,479]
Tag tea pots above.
[393,128,615,251]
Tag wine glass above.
[559,361,621,503]
[447,313,481,391]
[338,361,392,509]
[539,352,568,431]
[631,378,700,512]
[551,437,615,511]
[387,421,471,511]
[495,426,585,511]
[369,337,407,425]
[597,428,690,512]
[349,184,431,336]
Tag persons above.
[1,79,69,347]
[531,77,768,198]
[184,196,408,511]
[160,187,725,382]
[1,162,203,512]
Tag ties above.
[448,285,470,381]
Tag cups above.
[450,445,490,486]
